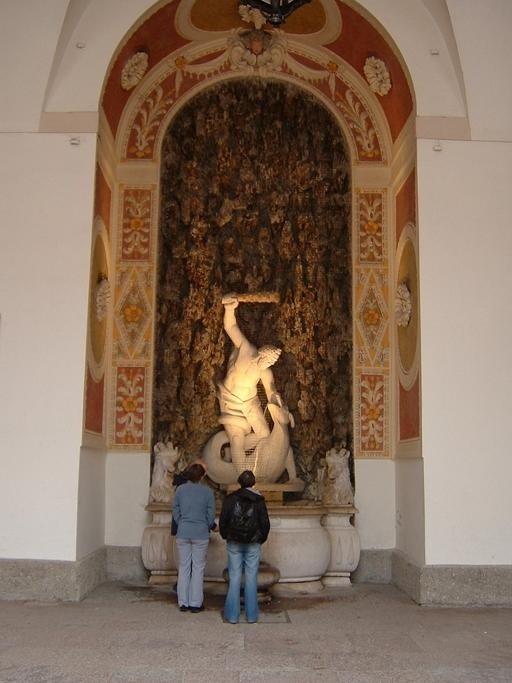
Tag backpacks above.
[228,499,256,542]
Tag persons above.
[321,446,355,505]
[213,288,289,478]
[169,458,219,595]
[216,468,272,624]
[150,438,180,505]
[169,463,218,613]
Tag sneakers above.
[180,603,204,613]
[229,619,257,624]
